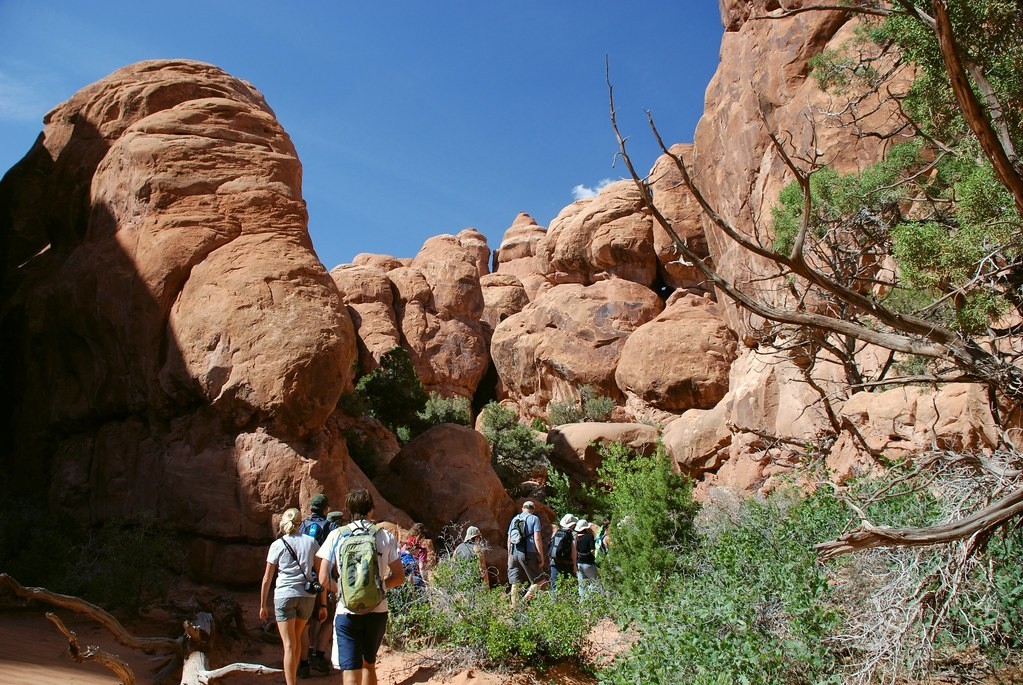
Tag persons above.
[375,521,435,605]
[507,501,550,608]
[298,494,348,680]
[314,488,406,685]
[451,526,490,621]
[259,508,327,685]
[551,514,611,600]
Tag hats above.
[309,494,328,513]
[463,526,482,543]
[575,520,590,532]
[326,511,343,522]
[523,501,535,510]
[559,513,577,528]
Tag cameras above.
[304,581,324,594]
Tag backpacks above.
[511,514,533,545]
[338,523,382,615]
[398,552,421,587]
[548,528,573,561]
[595,534,610,566]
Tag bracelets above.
[320,604,327,608]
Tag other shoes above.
[310,658,329,672]
[521,599,531,612]
[297,664,311,678]
